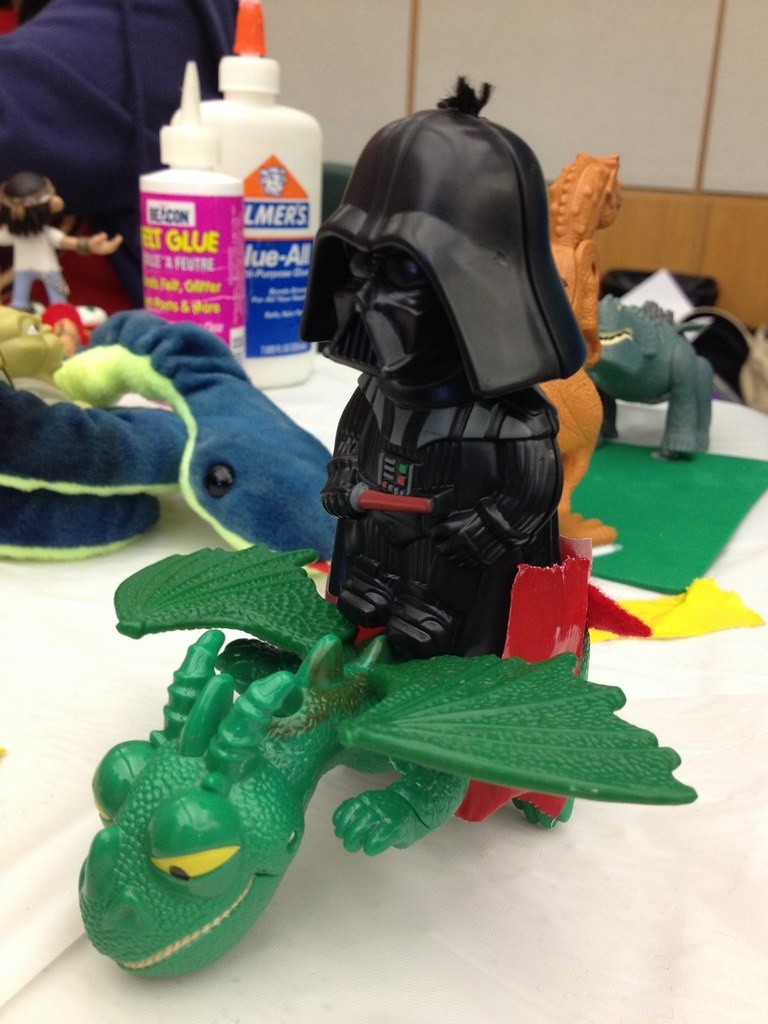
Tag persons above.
[1,0,237,301]
[297,73,587,657]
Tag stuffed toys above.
[1,308,344,579]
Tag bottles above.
[202,0,324,388]
[138,60,246,378]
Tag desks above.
[0,338,767,1024]
